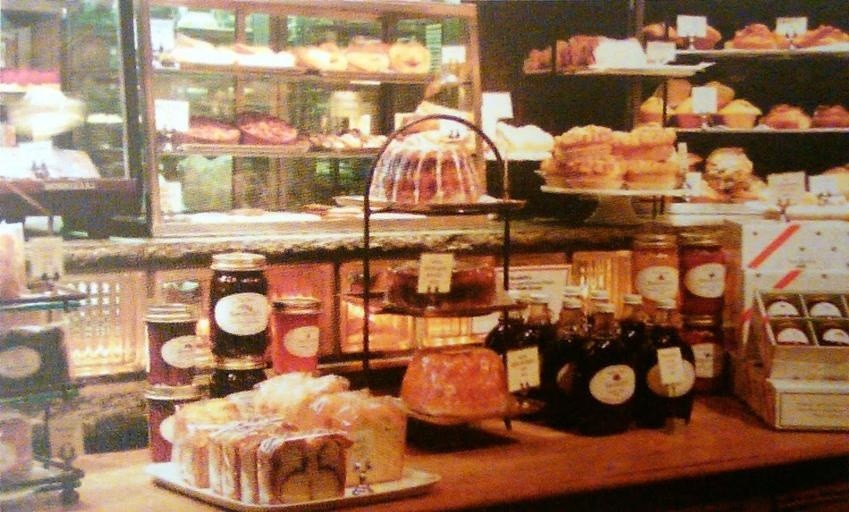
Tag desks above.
[0,394,849,512]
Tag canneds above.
[630,229,728,392]
[765,293,849,346]
[2,418,32,486]
[143,247,325,463]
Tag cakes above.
[535,123,686,191]
[638,77,848,130]
[523,35,649,69]
[643,19,848,49]
[688,145,849,206]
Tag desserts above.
[172,100,473,149]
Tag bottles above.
[488,287,697,439]
[207,252,271,359]
[763,294,848,348]
[271,296,324,373]
[144,386,200,464]
[141,302,200,386]
[629,232,685,319]
[681,314,726,393]
[679,229,729,317]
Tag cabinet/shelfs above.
[0,1,849,434]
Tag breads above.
[170,371,409,505]
[160,33,432,73]
[1,326,75,394]
[0,223,30,299]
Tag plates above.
[185,109,299,145]
